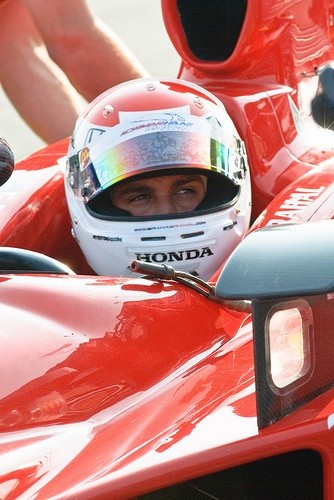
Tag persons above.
[61,75,252,283]
[0,0,143,146]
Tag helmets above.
[65,78,254,283]
